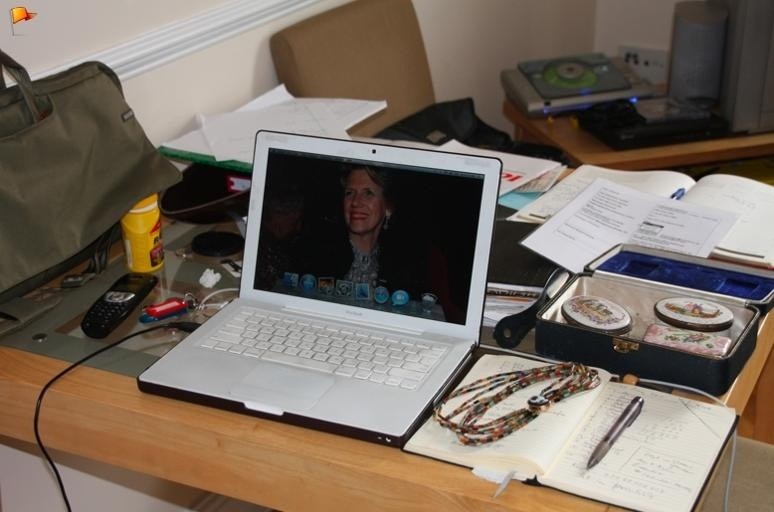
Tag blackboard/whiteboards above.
[3,2,306,83]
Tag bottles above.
[560,295,632,338]
[121,195,164,272]
[653,295,732,358]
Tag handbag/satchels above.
[0,49,182,305]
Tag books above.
[402,354,739,512]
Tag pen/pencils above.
[670,188,685,201]
[585,395,644,468]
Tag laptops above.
[136,129,503,448]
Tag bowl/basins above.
[157,164,249,223]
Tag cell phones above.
[81,271,159,338]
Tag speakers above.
[666,3,728,102]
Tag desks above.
[498,81,773,187]
[0,208,774,510]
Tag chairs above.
[264,0,506,153]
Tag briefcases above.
[535,243,774,396]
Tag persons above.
[299,163,413,297]
